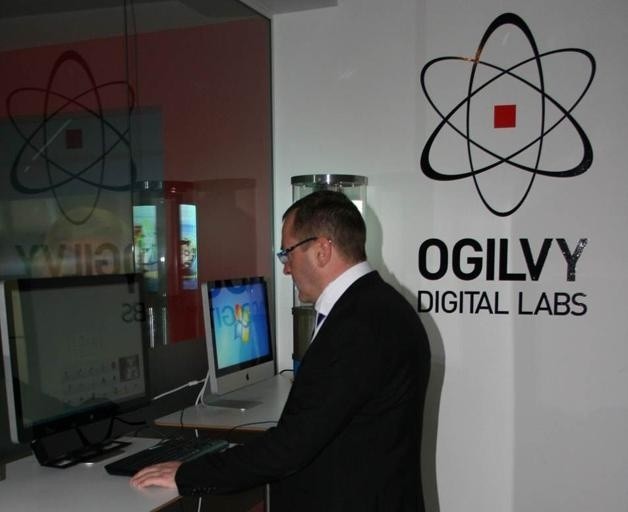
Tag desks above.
[0,373,294,511]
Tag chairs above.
[175,438,286,512]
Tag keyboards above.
[104,433,229,477]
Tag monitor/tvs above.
[199,275,277,411]
[0,272,152,469]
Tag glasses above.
[277,237,333,264]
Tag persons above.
[131,191,431,512]
